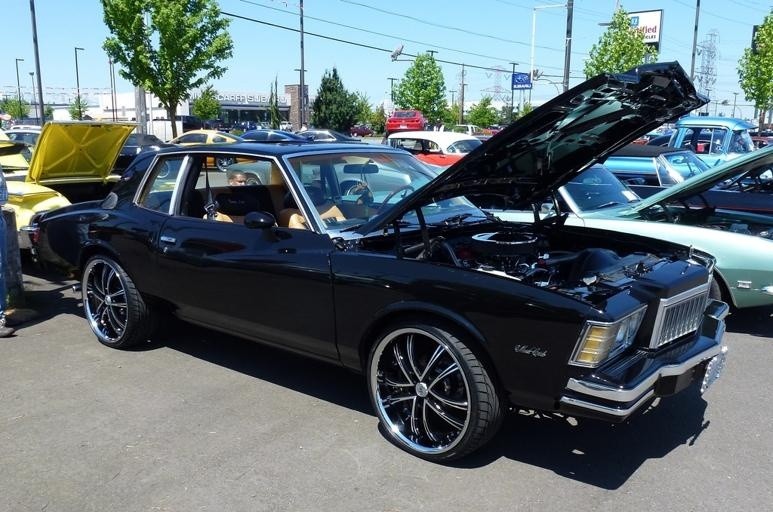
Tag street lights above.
[15,58,25,125]
[74,47,85,120]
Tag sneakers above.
[1,326,15,337]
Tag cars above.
[32,60,730,464]
[352,126,374,138]
[0,119,184,251]
[166,121,362,172]
[596,147,749,198]
[478,140,773,331]
[646,118,773,181]
[382,110,493,177]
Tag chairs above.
[202,186,346,231]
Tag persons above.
[0,165,14,340]
[228,168,249,186]
